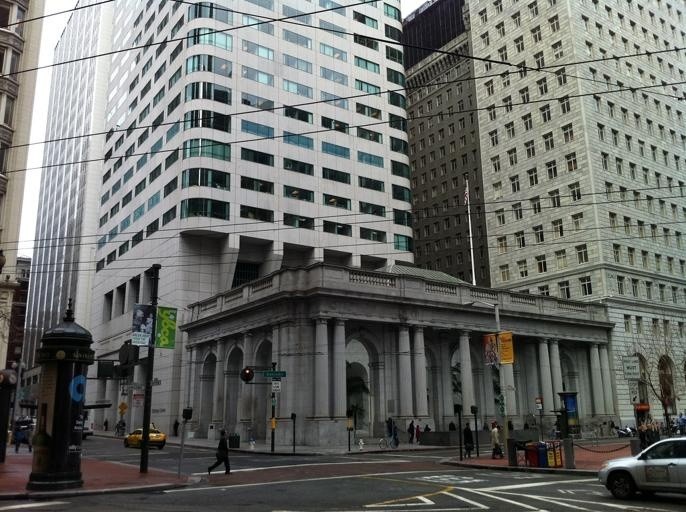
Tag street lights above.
[9,361,26,432]
[466,297,508,455]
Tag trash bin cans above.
[630,439,641,455]
[229,432,240,448]
[208,423,216,440]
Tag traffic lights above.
[244,368,250,380]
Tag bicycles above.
[377,435,398,449]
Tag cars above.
[122,427,166,450]
[597,434,686,501]
[13,415,94,441]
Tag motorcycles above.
[615,424,634,437]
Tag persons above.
[600,412,686,451]
[144,312,153,336]
[103,418,108,431]
[14,426,24,452]
[385,416,537,460]
[172,419,180,436]
[207,430,232,477]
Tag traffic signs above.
[263,371,286,377]
[272,378,281,392]
